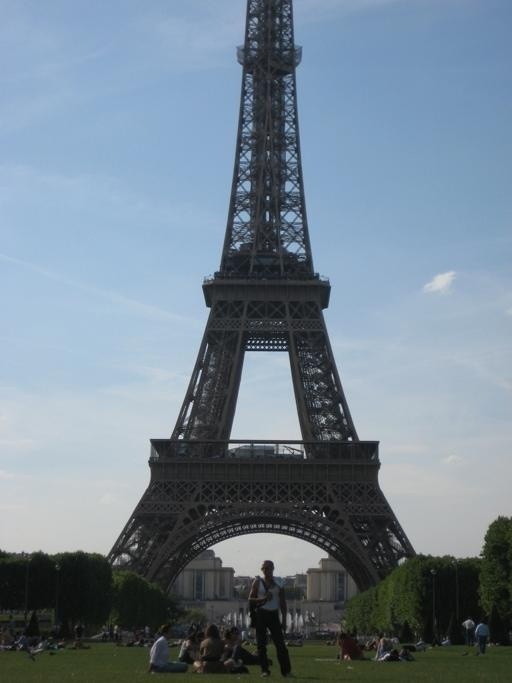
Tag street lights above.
[53,562,62,640]
[451,558,464,622]
[430,568,437,637]
[23,550,34,637]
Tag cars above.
[286,631,302,640]
[92,630,114,641]
[312,630,333,639]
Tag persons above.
[337,632,451,662]
[474,618,490,655]
[462,615,476,646]
[248,560,291,678]
[0,615,273,674]
